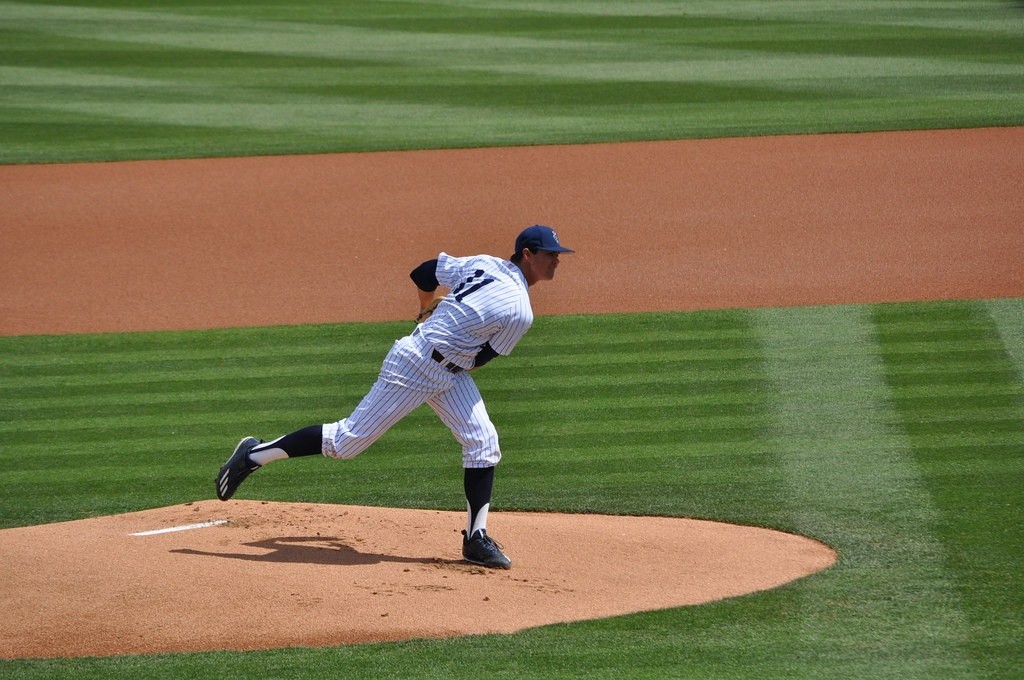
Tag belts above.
[431,348,464,375]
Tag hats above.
[515,224,575,254]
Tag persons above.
[215,217,569,573]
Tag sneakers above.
[215,435,267,501]
[460,528,512,569]
